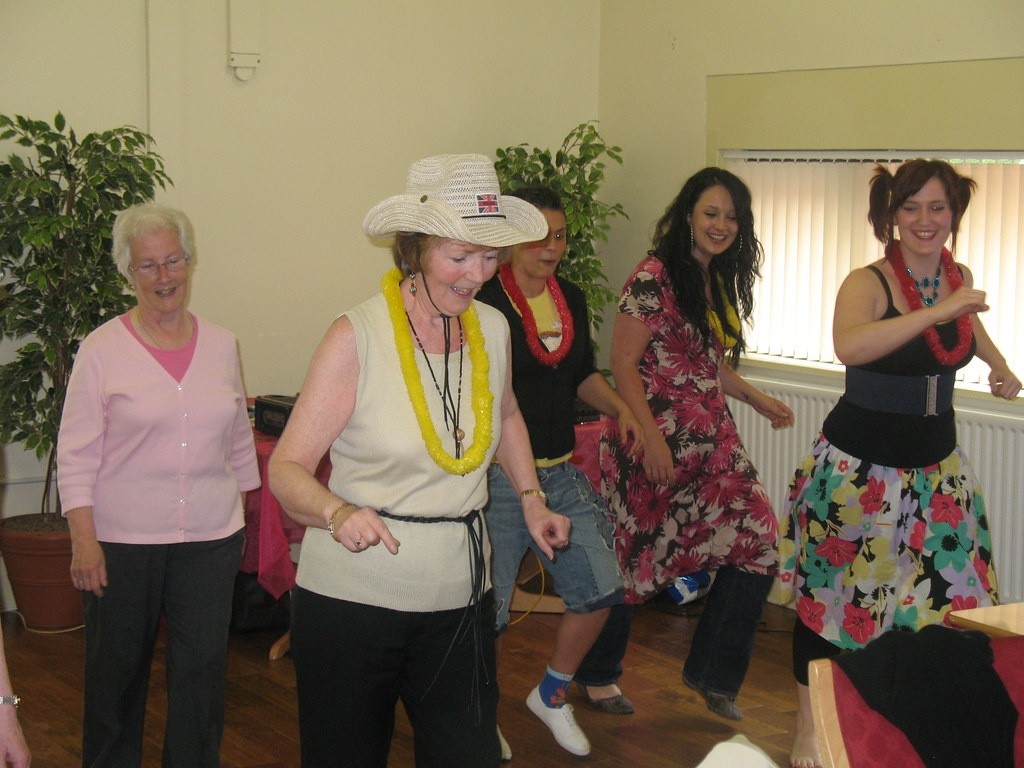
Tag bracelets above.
[329,503,358,543]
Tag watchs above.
[520,489,547,504]
[0,694,21,709]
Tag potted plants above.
[0,111,175,629]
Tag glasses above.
[128,252,189,279]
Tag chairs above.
[808,636,1024,768]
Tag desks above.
[242,398,623,662]
[949,602,1024,636]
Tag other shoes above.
[573,673,634,714]
[682,663,744,722]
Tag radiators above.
[725,376,1024,605]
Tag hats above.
[360,154,550,248]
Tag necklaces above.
[380,267,494,475]
[884,239,972,367]
[398,281,465,461]
[499,258,574,365]
[705,271,740,348]
[136,305,193,350]
[906,262,941,307]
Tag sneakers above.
[496,722,512,760]
[525,684,590,757]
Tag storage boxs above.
[255,395,298,437]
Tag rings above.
[355,535,362,547]
[78,580,83,584]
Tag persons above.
[267,151,571,768]
[57,201,262,768]
[789,160,1022,768]
[571,167,795,721]
[0,615,32,768]
[473,187,647,759]
